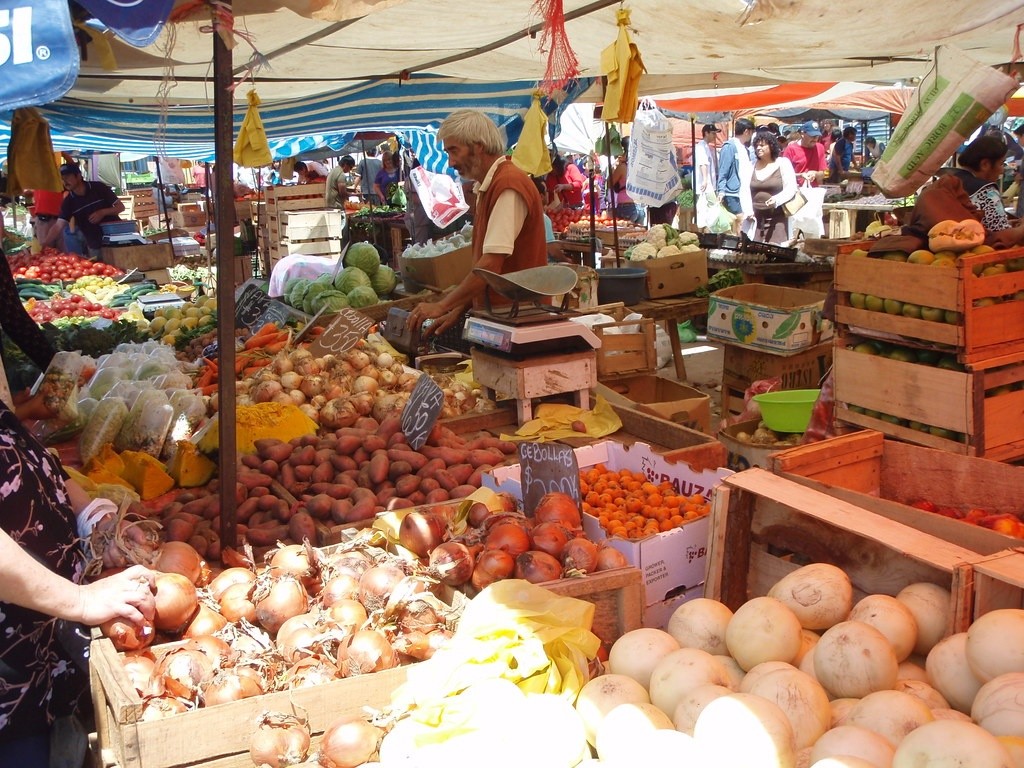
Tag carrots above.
[193,323,378,395]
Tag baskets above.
[100,220,137,235]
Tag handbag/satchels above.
[624,100,684,209]
[787,180,827,241]
[740,215,758,242]
[409,165,471,229]
[570,202,585,210]
[781,189,808,217]
[694,193,742,234]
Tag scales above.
[460,267,604,363]
[137,293,186,316]
[101,233,148,246]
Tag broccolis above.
[630,224,701,267]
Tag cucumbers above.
[14,278,53,300]
[110,283,157,307]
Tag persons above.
[716,118,756,248]
[740,132,796,245]
[783,120,829,239]
[152,134,693,233]
[0,151,90,259]
[39,162,125,260]
[0,397,158,768]
[936,135,1024,247]
[946,120,1024,219]
[692,124,717,249]
[0,211,70,406]
[406,108,551,338]
[746,119,886,183]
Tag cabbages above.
[284,242,396,314]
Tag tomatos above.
[28,294,126,324]
[6,247,124,284]
[546,207,591,232]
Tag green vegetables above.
[695,267,746,298]
[54,310,219,351]
[354,184,406,230]
[676,187,694,208]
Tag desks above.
[601,246,830,284]
[631,294,709,380]
[554,238,599,269]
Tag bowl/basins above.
[594,268,648,307]
[752,389,821,433]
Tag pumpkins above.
[84,402,318,500]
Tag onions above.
[736,420,802,447]
[136,294,217,345]
[201,346,497,426]
[565,210,629,229]
[100,491,625,768]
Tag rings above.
[414,314,418,319]
[135,582,141,591]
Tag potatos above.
[117,414,516,560]
[572,420,586,432]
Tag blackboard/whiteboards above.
[234,284,292,339]
[519,442,583,522]
[400,374,444,449]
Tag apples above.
[909,497,1024,544]
[846,246,1024,445]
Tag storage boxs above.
[0,184,1024,768]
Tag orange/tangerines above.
[581,463,711,537]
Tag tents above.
[636,81,921,168]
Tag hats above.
[799,120,823,137]
[767,122,781,136]
[60,162,81,174]
[701,124,722,133]
[776,135,788,143]
